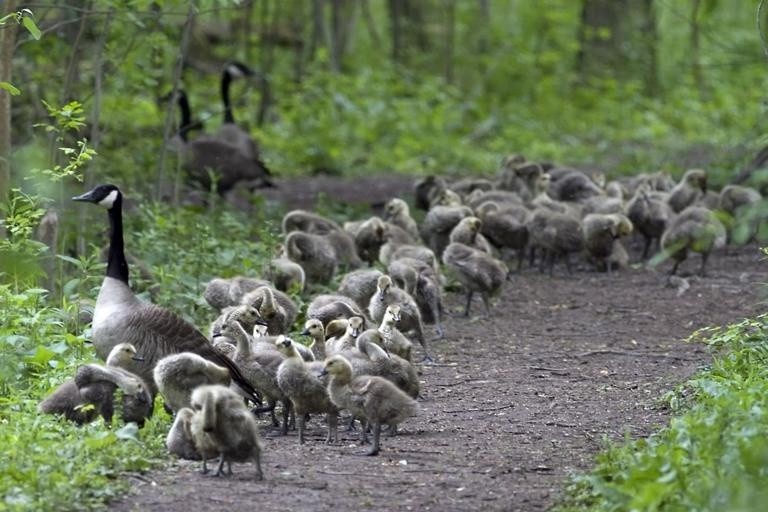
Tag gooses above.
[160,86,269,194]
[215,59,271,196]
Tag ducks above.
[41,342,261,479]
[204,198,440,459]
[71,181,261,422]
[412,151,766,278]
[440,217,507,321]
